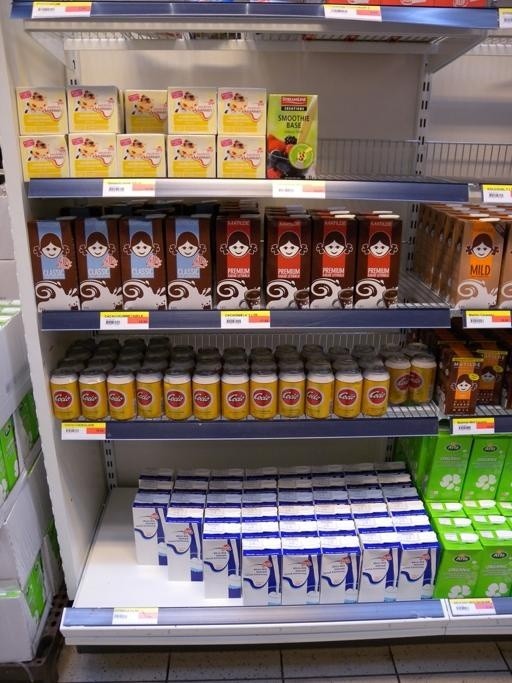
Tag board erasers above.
[289,144,313,169]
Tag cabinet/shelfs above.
[3,4,512,185]
[40,315,512,435]
[57,435,512,645]
[23,184,512,315]
